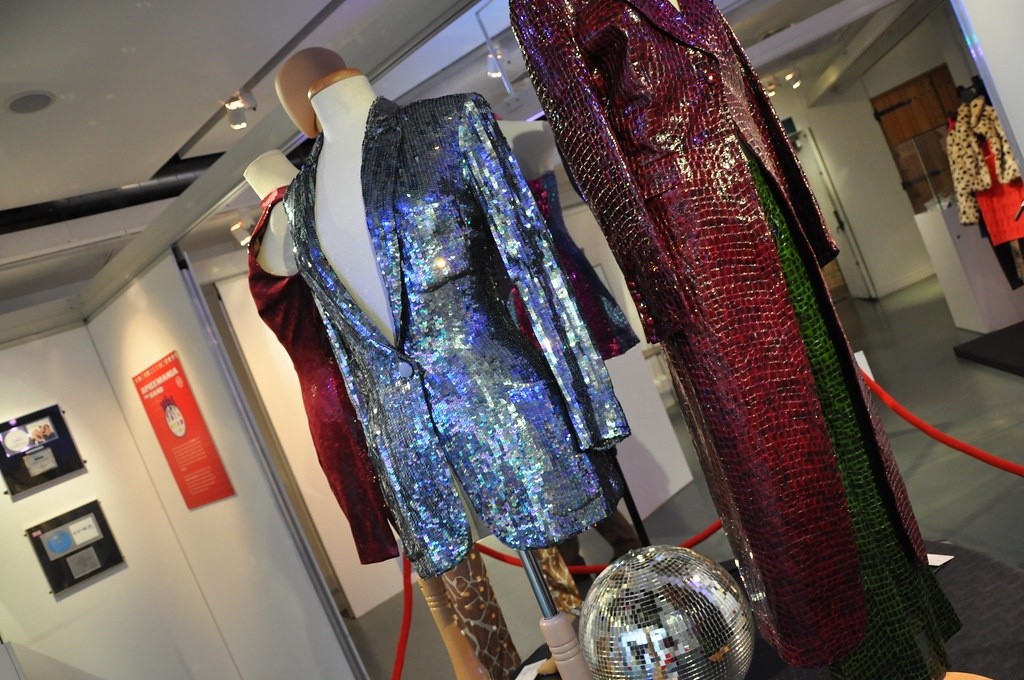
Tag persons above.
[558,510,639,581]
[243,149,400,565]
[31,424,56,444]
[283,68,632,582]
[508,0,964,680]
